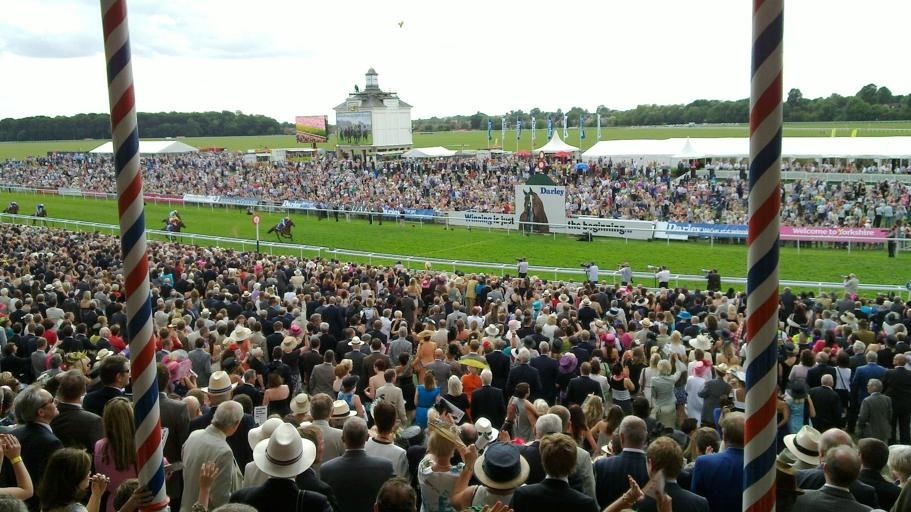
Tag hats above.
[281,324,302,352]
[484,323,499,336]
[95,348,114,361]
[223,325,251,349]
[589,317,616,346]
[676,310,691,319]
[712,363,727,374]
[200,370,360,479]
[688,327,731,350]
[639,318,654,327]
[783,425,822,465]
[840,311,858,324]
[44,284,55,291]
[473,417,530,489]
[557,352,578,374]
[348,336,365,347]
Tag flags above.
[580,114,585,139]
[597,114,600,139]
[501,118,507,141]
[548,115,552,138]
[564,116,568,138]
[531,117,536,139]
[517,116,521,140]
[488,120,491,141]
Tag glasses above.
[38,398,54,409]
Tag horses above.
[3,204,19,214]
[267,219,296,243]
[162,213,182,225]
[519,187,549,233]
[29,209,48,226]
[161,221,186,242]
[363,130,368,142]
[340,128,362,144]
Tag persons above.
[0,148,910,512]
[297,131,326,142]
[339,124,368,144]
